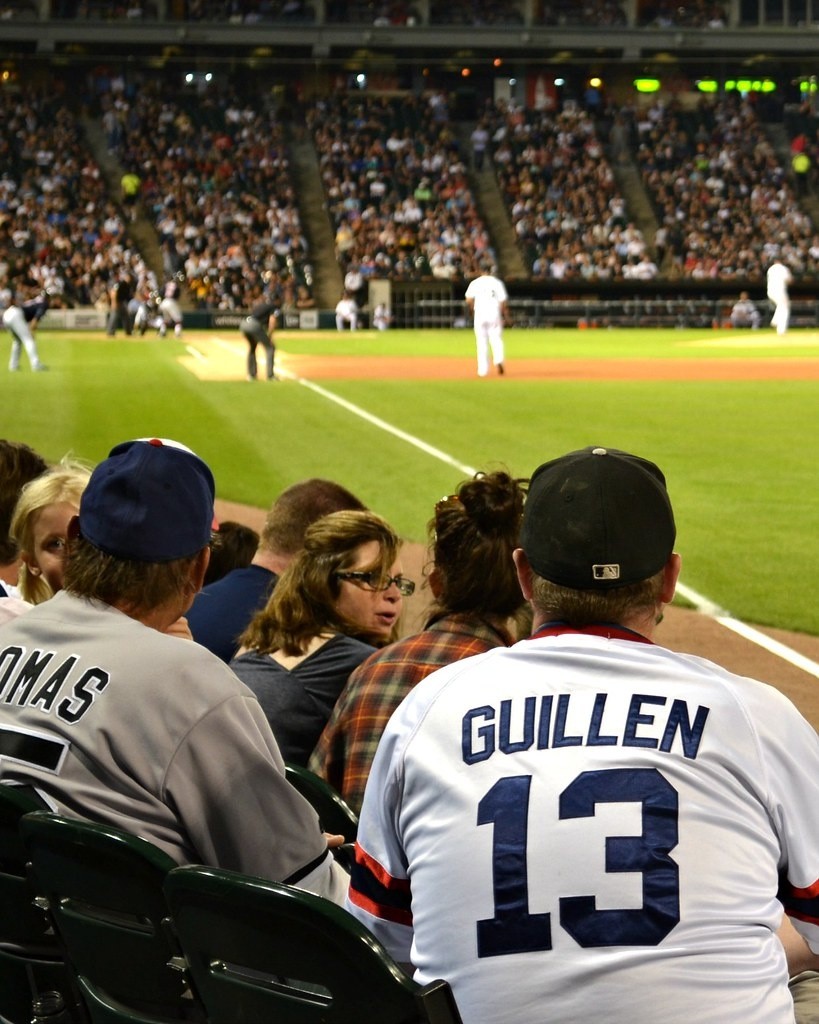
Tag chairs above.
[0,763,462,1024]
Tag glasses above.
[334,572,415,596]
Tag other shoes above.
[498,364,504,374]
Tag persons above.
[183,480,369,667]
[242,293,284,382]
[0,438,192,642]
[0,88,819,373]
[311,472,531,819]
[232,510,403,770]
[466,265,512,376]
[0,438,350,907]
[348,446,818,1023]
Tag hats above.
[79,437,215,561]
[518,445,677,593]
